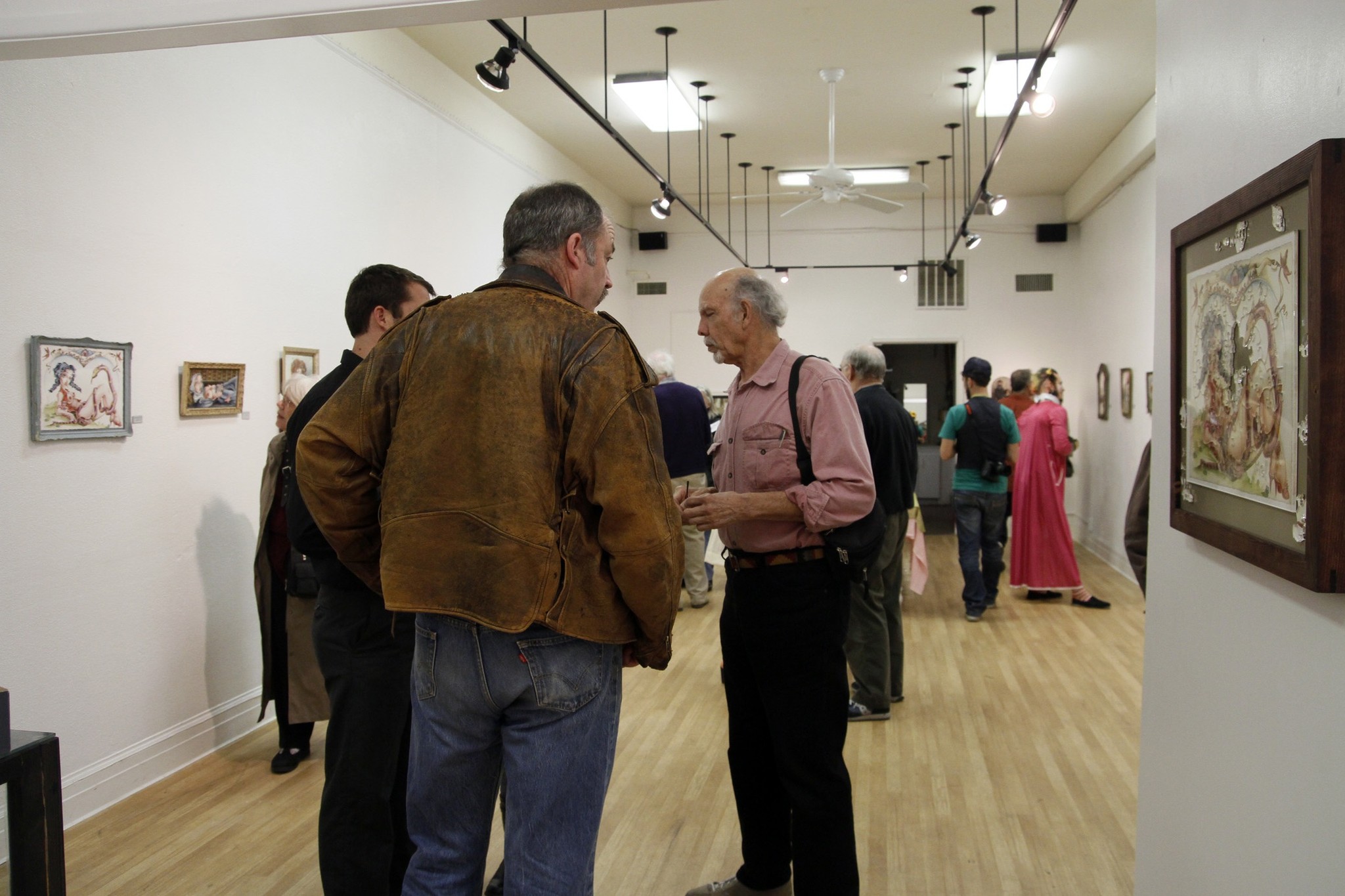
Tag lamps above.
[972,49,1061,123]
[979,189,1010,217]
[958,226,983,252]
[611,71,704,138]
[892,268,910,284]
[776,271,790,282]
[648,183,678,223]
[775,165,912,188]
[939,261,958,280]
[1018,83,1061,127]
[470,44,520,95]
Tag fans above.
[731,66,906,222]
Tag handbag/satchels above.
[788,355,888,581]
[285,545,321,599]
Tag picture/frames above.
[25,331,140,445]
[1119,367,1135,420]
[1096,363,1111,420]
[1145,371,1153,415]
[277,344,322,396]
[1166,136,1345,598]
[177,358,248,419]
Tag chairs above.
[0,684,68,896]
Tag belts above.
[728,547,825,571]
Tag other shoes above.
[685,875,792,896]
[965,612,980,621]
[848,699,890,721]
[678,608,683,611]
[1073,596,1110,608]
[271,742,310,774]
[986,602,994,608]
[708,580,713,591]
[851,682,903,702]
[692,598,709,608]
[1027,590,1062,599]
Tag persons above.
[674,267,876,896]
[695,385,724,592]
[992,369,1034,572]
[254,375,333,773]
[297,181,686,896]
[263,265,437,896]
[1124,439,1152,597]
[841,345,918,721]
[1010,367,1110,607]
[648,351,711,611]
[939,357,1020,621]
